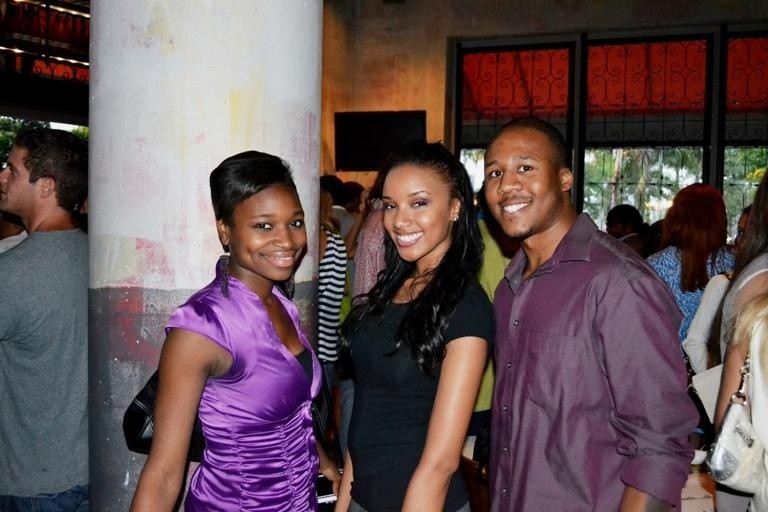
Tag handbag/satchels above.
[122,367,340,462]
[709,404,765,495]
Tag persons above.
[605,183,756,458]
[319,172,388,454]
[485,113,704,511]
[127,151,344,511]
[469,186,522,444]
[699,168,768,511]
[334,143,495,511]
[0,126,93,511]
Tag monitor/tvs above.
[334,109,427,173]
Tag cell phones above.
[315,474,338,504]
